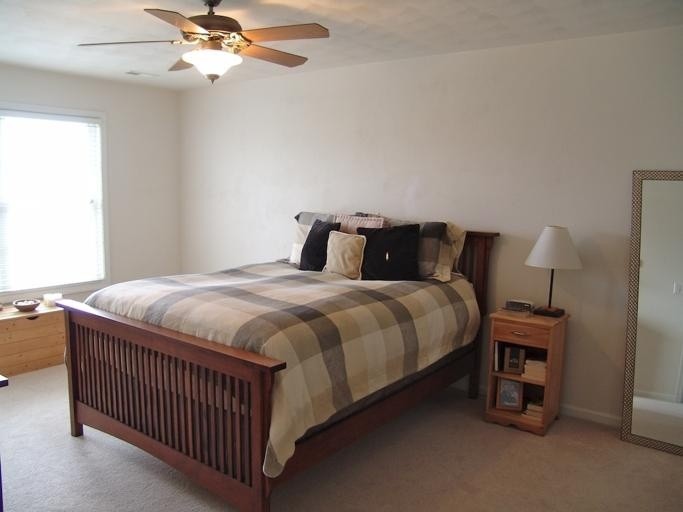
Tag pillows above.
[290,209,447,283]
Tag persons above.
[509,383,519,406]
[500,383,512,405]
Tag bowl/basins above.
[12,298,41,313]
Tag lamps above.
[180,49,242,83]
[524,226,584,319]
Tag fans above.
[76,0,329,73]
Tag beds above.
[57,210,499,512]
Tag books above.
[519,359,547,423]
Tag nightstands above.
[486,310,572,435]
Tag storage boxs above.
[0,302,65,378]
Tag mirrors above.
[619,168,683,456]
